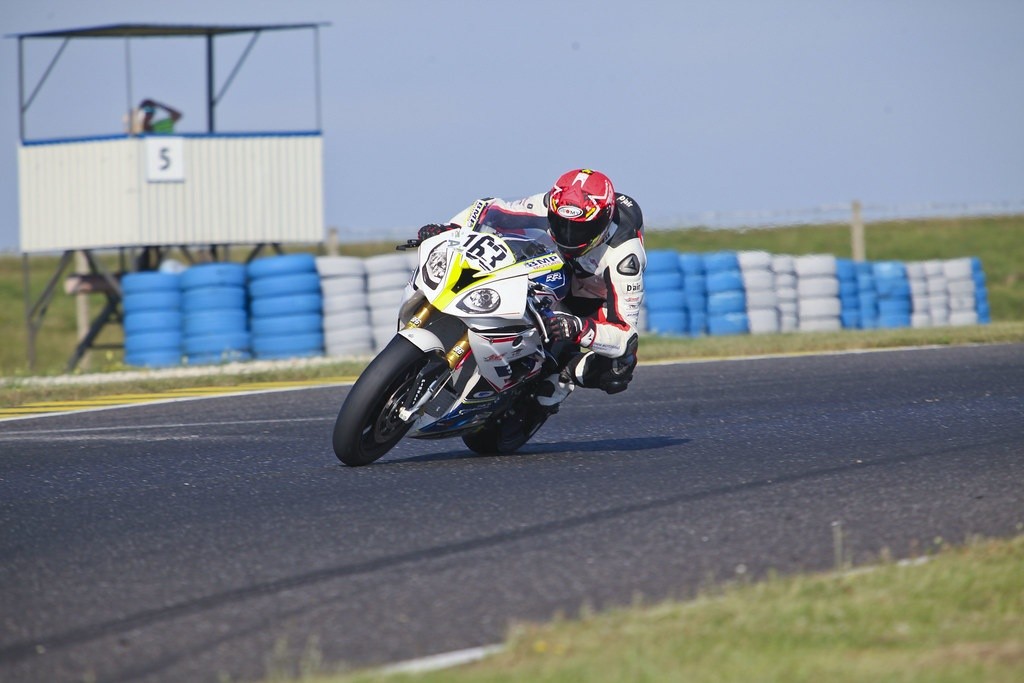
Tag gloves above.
[418,222,454,241]
[543,312,584,341]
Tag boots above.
[536,349,595,407]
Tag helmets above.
[547,170,616,259]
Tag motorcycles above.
[332,226,568,467]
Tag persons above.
[120,96,183,135]
[416,167,648,395]
